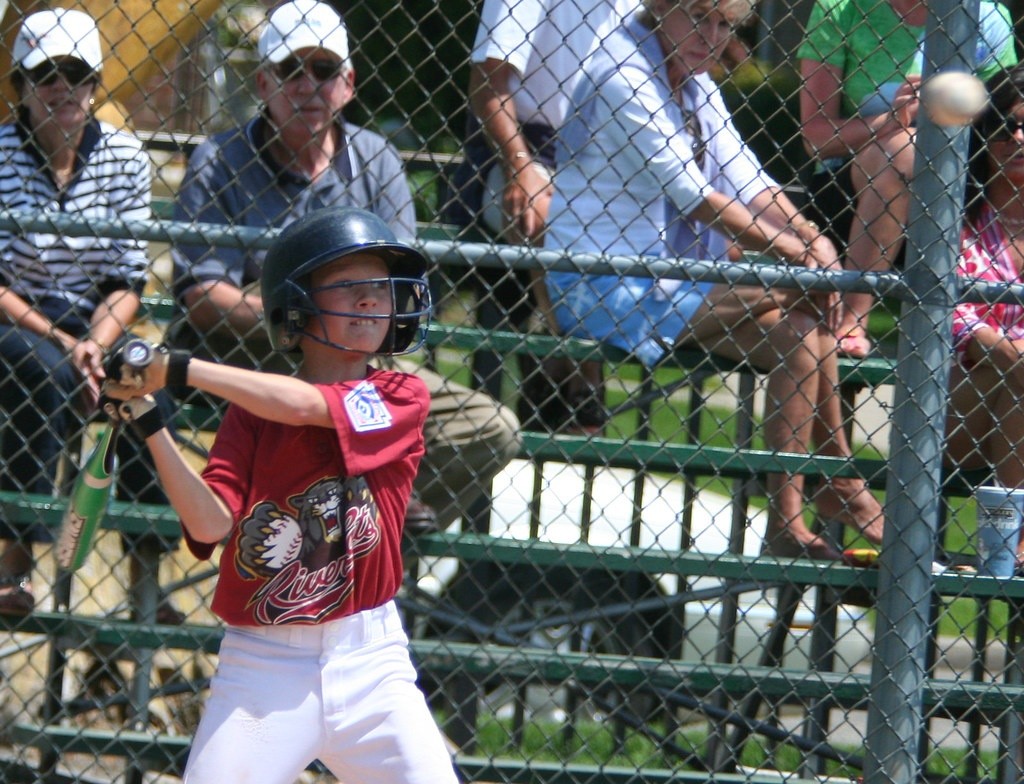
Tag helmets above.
[261,205,431,357]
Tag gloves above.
[97,334,194,401]
[97,378,167,441]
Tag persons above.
[0,6,185,626]
[103,205,458,784]
[169,0,516,562]
[453,0,1024,566]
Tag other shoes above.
[129,600,186,626]
[0,581,35,618]
[402,490,437,534]
[834,334,870,358]
[562,390,604,434]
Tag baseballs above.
[924,70,988,126]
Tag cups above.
[973,485,1024,579]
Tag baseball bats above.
[54,337,153,575]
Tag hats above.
[257,0,348,64]
[11,8,104,73]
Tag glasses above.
[682,109,706,173]
[20,58,94,86]
[981,114,1024,142]
[270,58,347,82]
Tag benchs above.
[1,200,1024,784]
